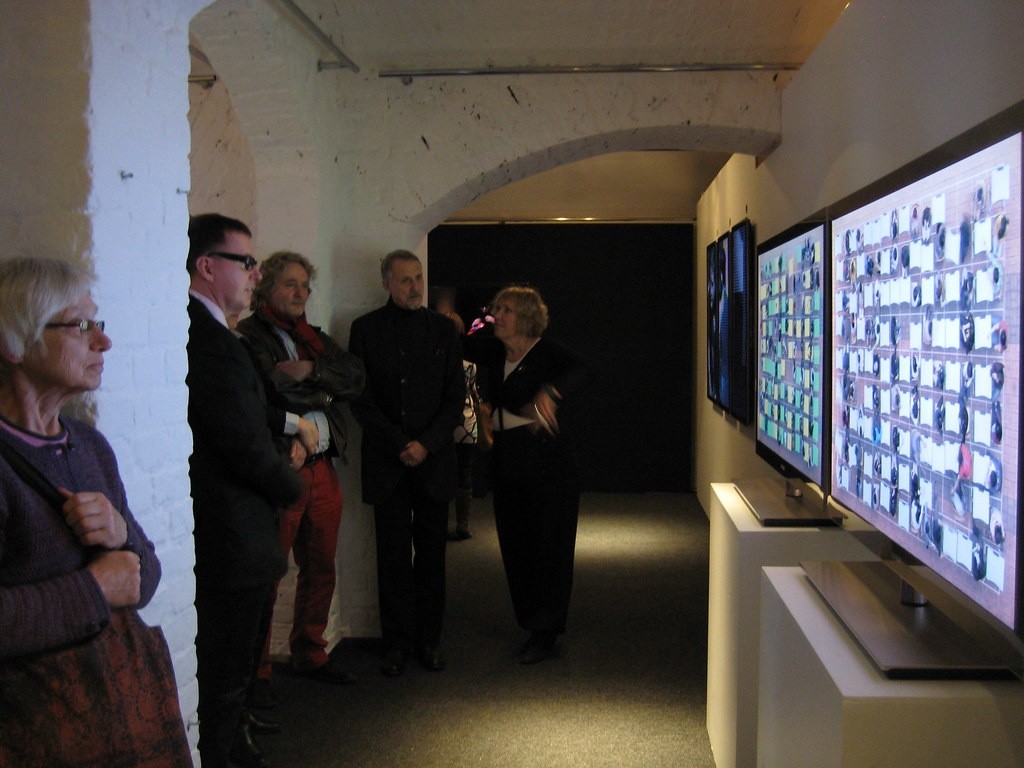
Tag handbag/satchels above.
[0,442,194,768]
[475,403,494,449]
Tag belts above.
[303,448,332,466]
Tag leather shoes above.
[227,724,271,768]
[248,713,282,733]
[415,642,445,671]
[385,647,405,677]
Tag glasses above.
[44,318,105,340]
[207,252,258,272]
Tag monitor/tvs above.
[731,206,848,524]
[796,97,1024,678]
[705,214,755,427]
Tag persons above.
[348,249,467,674]
[0,256,162,660]
[435,308,491,538]
[481,287,583,665]
[187,214,304,768]
[235,251,366,730]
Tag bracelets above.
[545,384,561,406]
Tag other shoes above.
[293,664,359,684]
[514,633,560,663]
[456,524,472,540]
[248,673,281,708]
